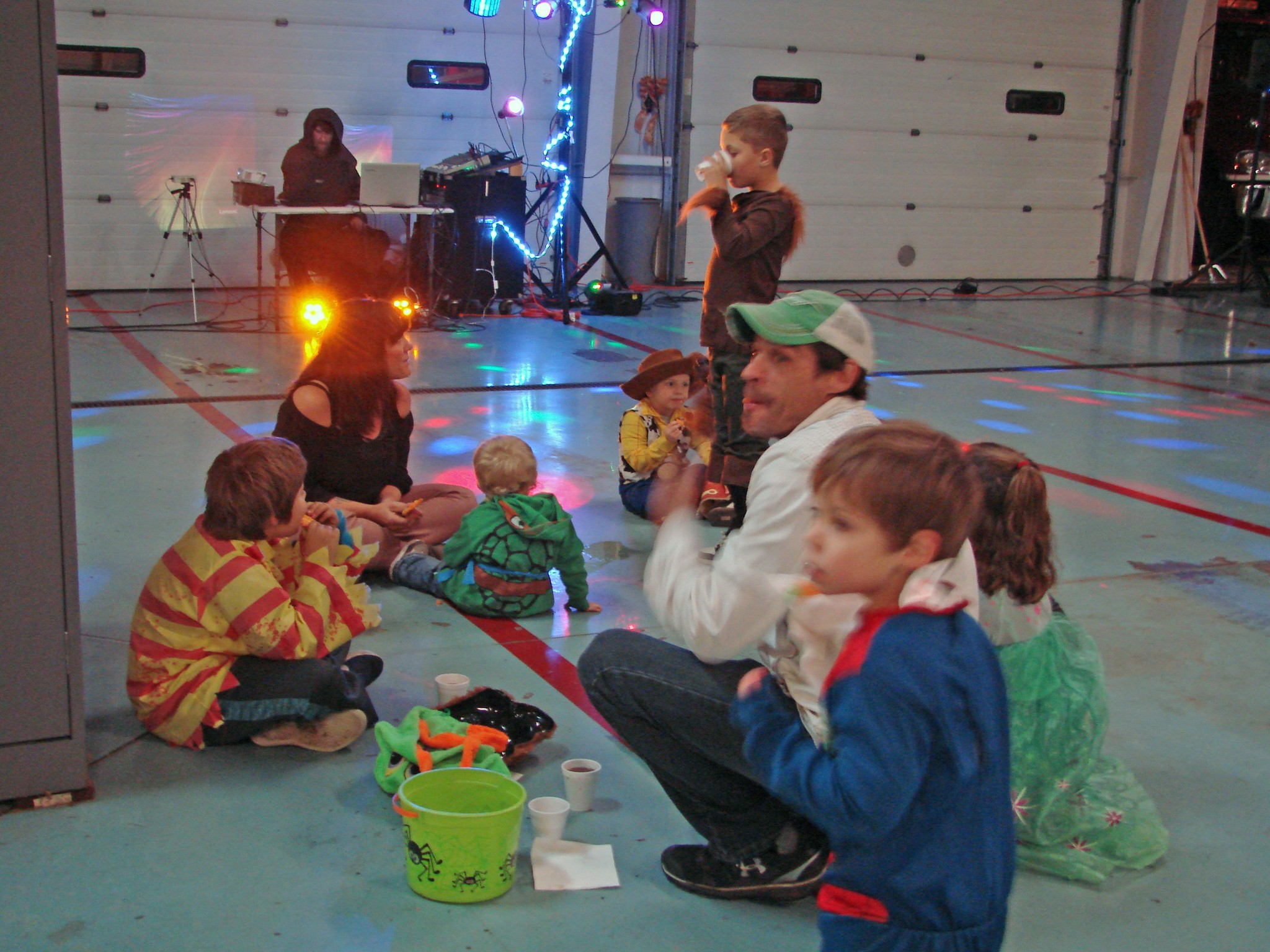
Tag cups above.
[529,796,570,841]
[561,758,601,812]
[435,673,470,705]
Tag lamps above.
[531,0,560,20]
[602,0,625,8]
[498,96,525,162]
[463,0,501,17]
[630,0,664,26]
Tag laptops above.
[349,162,420,206]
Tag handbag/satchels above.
[375,705,512,795]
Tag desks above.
[250,204,455,332]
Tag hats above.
[619,349,710,400]
[725,288,875,372]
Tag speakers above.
[1248,39,1270,91]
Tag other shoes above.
[707,506,735,527]
[432,543,445,561]
[249,707,366,752]
[389,540,429,580]
[341,650,384,687]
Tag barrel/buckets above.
[392,767,528,903]
[605,196,663,287]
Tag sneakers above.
[659,820,831,901]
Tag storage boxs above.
[231,180,276,207]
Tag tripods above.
[138,182,223,323]
[1176,91,1270,295]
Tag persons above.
[617,347,736,527]
[575,290,882,900]
[730,418,1018,952]
[271,297,478,573]
[277,108,391,285]
[388,435,603,619]
[125,437,383,751]
[677,104,807,552]
[963,442,1167,880]
